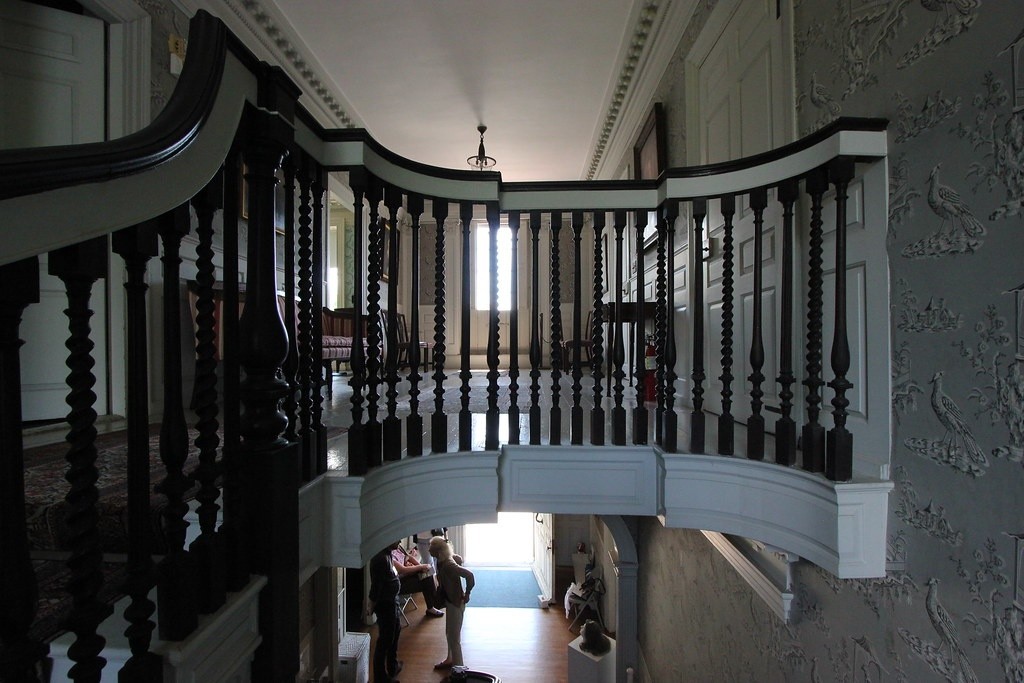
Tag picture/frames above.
[380,217,401,286]
[634,102,669,254]
[241,164,285,236]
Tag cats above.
[579,619,612,657]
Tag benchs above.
[187,278,384,386]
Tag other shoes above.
[426,607,444,617]
[373,678,400,683]
[434,660,453,670]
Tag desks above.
[600,301,657,397]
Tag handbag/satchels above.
[580,577,606,601]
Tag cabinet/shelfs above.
[589,514,619,632]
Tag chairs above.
[398,594,418,626]
[561,311,594,376]
[566,564,604,632]
[380,310,435,373]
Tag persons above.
[428,536,475,670]
[391,545,445,617]
[368,540,404,683]
[579,619,601,643]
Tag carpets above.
[460,569,551,609]
